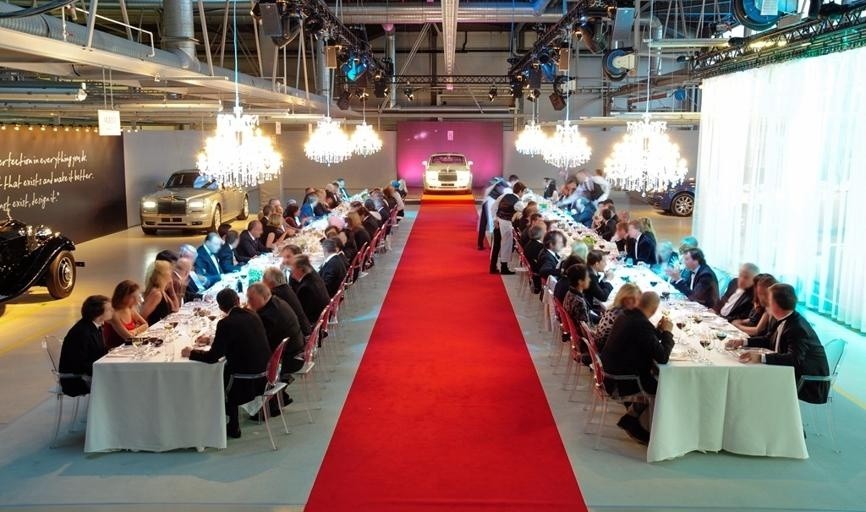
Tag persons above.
[59,180,410,438]
[478,169,830,446]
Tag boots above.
[501,261,516,275]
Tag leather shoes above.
[225,421,242,439]
[615,413,650,444]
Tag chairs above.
[44,182,403,466]
[488,182,848,464]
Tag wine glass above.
[550,205,730,363]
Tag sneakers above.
[489,266,499,273]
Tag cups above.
[132,219,319,360]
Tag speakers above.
[326,46,337,68]
[614,8,635,42]
[559,48,570,72]
[259,3,282,38]
[375,81,384,97]
[530,69,540,87]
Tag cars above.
[139,168,250,235]
[647,177,697,217]
[422,153,474,194]
[0,218,85,318]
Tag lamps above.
[600,1,694,197]
[192,2,290,189]
[513,86,552,162]
[349,81,384,159]
[540,30,595,172]
[303,35,357,169]
[352,77,540,106]
